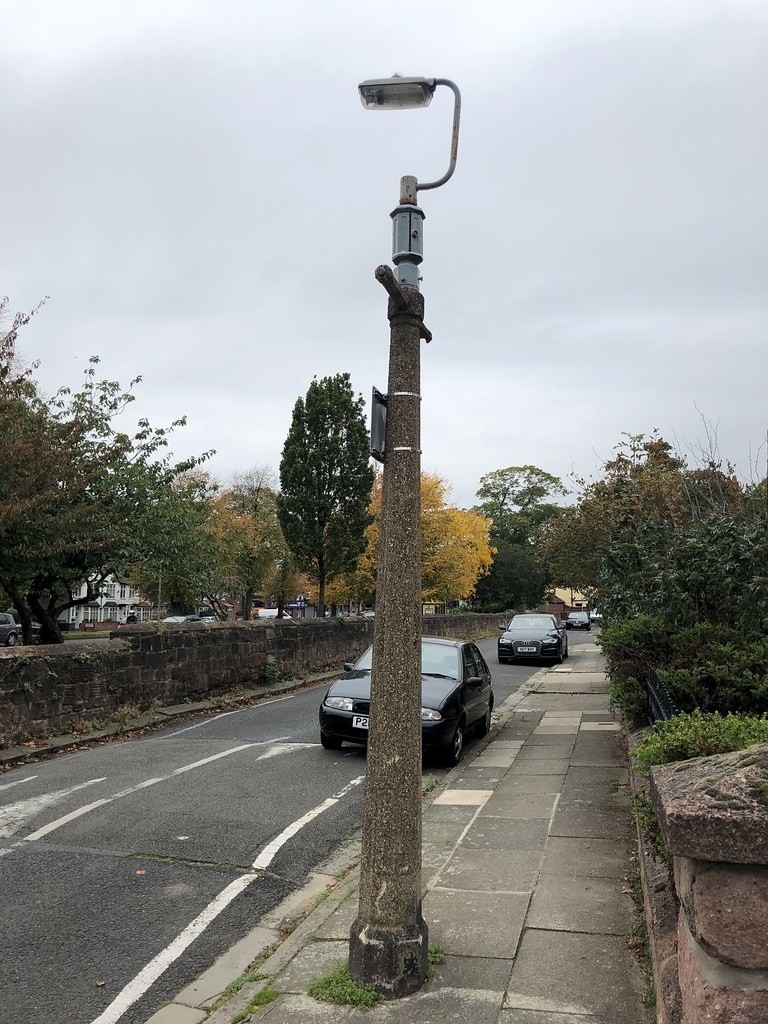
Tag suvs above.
[0,613,17,646]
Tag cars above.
[339,611,375,617]
[162,615,202,623]
[201,615,216,622]
[17,621,43,635]
[319,634,494,767]
[566,611,592,631]
[590,608,603,623]
[498,614,569,665]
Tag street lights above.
[345,72,464,998]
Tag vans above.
[250,607,293,620]
